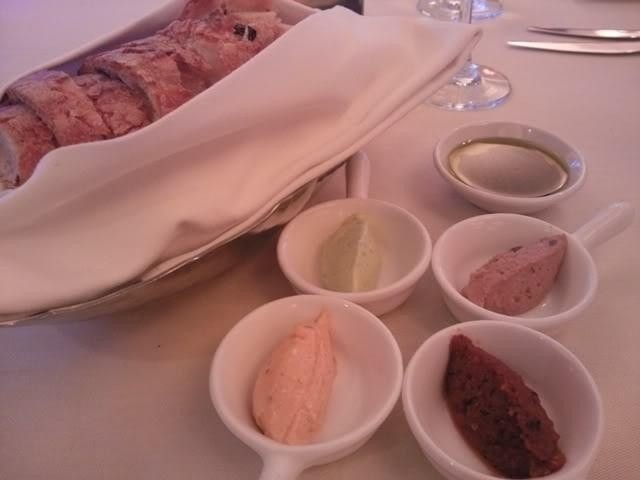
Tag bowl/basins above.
[431,212,599,339]
[433,118,589,212]
[275,149,432,316]
[207,292,403,480]
[402,319,605,480]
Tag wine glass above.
[417,1,512,110]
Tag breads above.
[0,0,283,191]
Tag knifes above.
[525,28,640,39]
[503,41,639,56]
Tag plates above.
[0,177,319,327]
